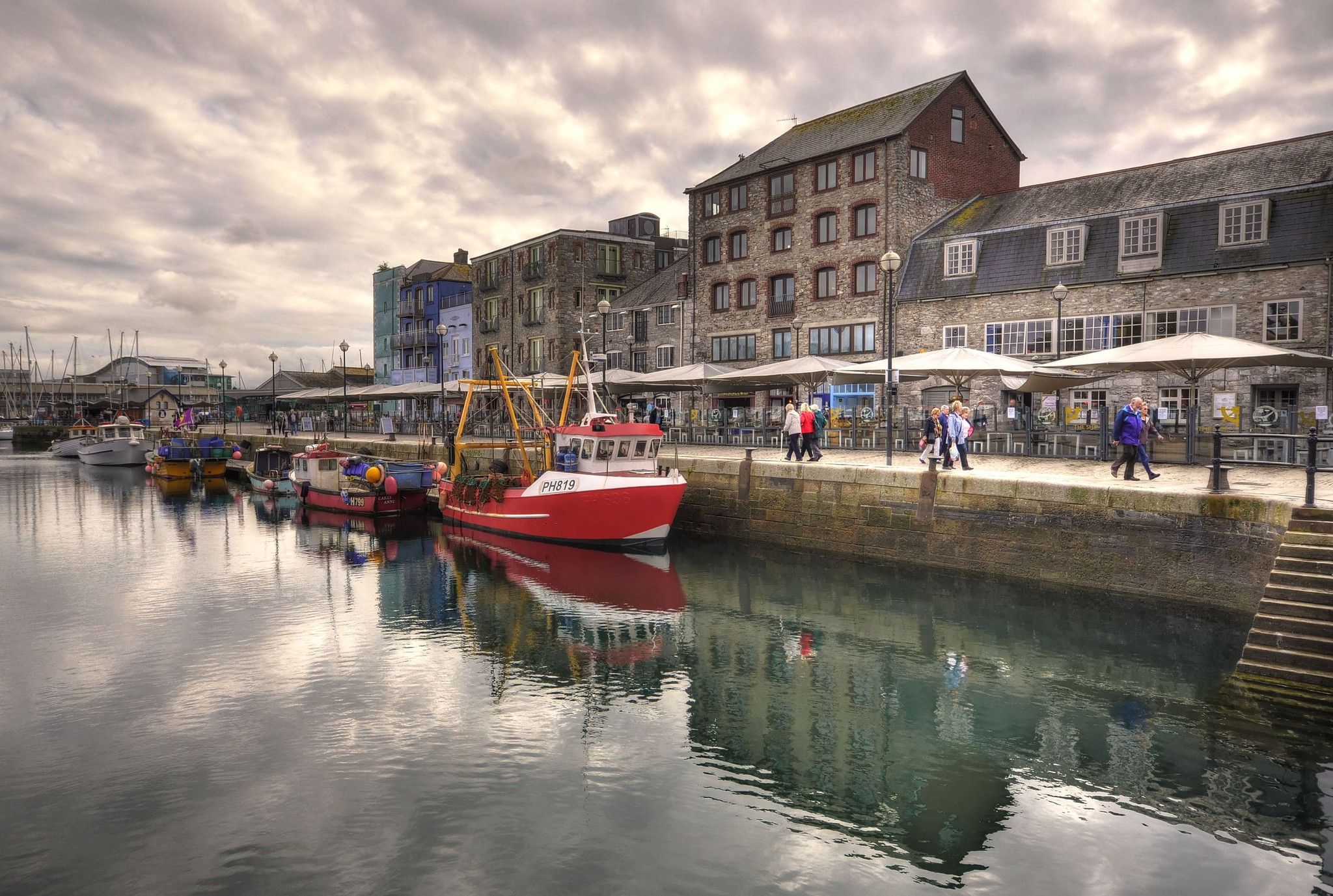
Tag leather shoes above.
[1110,467,1117,478]
[1124,476,1140,481]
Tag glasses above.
[1142,409,1148,410]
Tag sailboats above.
[0,324,160,468]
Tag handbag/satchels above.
[919,438,925,448]
[949,442,959,461]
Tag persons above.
[276,412,286,435]
[649,404,664,447]
[974,400,989,431]
[920,401,974,471]
[200,411,218,420]
[287,409,298,435]
[1111,397,1163,480]
[172,409,179,430]
[782,403,827,461]
[100,408,111,421]
[1009,399,1020,430]
[194,412,201,430]
[457,410,462,421]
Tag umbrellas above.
[1033,332,1333,407]
[707,355,929,404]
[831,346,1088,395]
[277,362,769,426]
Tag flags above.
[174,408,193,429]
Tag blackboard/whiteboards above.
[379,418,393,433]
[301,418,313,430]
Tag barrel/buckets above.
[282,469,290,477]
[158,437,242,459]
[421,468,432,487]
[342,463,372,480]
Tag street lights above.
[145,372,152,429]
[625,332,635,402]
[268,350,279,435]
[503,347,511,380]
[175,365,183,417]
[68,378,75,418]
[1050,280,1071,431]
[217,383,222,419]
[118,375,125,413]
[363,362,371,410]
[423,357,430,408]
[338,339,351,440]
[877,249,904,466]
[219,359,227,434]
[435,324,449,443]
[104,381,108,396]
[596,294,613,415]
[791,313,806,414]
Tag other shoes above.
[942,467,951,470]
[816,455,823,461]
[795,458,803,461]
[807,458,814,461]
[963,467,973,471]
[1149,473,1160,480]
[919,458,925,463]
[785,457,790,461]
[952,465,956,469]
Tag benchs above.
[972,438,1006,453]
[1014,440,1072,456]
[664,432,919,449]
[1082,442,1112,460]
[1297,446,1330,465]
[1234,445,1269,460]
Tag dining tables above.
[662,427,920,450]
[986,431,1112,457]
[1253,437,1333,465]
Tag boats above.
[246,417,297,495]
[287,439,448,518]
[434,519,692,682]
[288,501,428,566]
[143,407,242,479]
[426,343,691,552]
